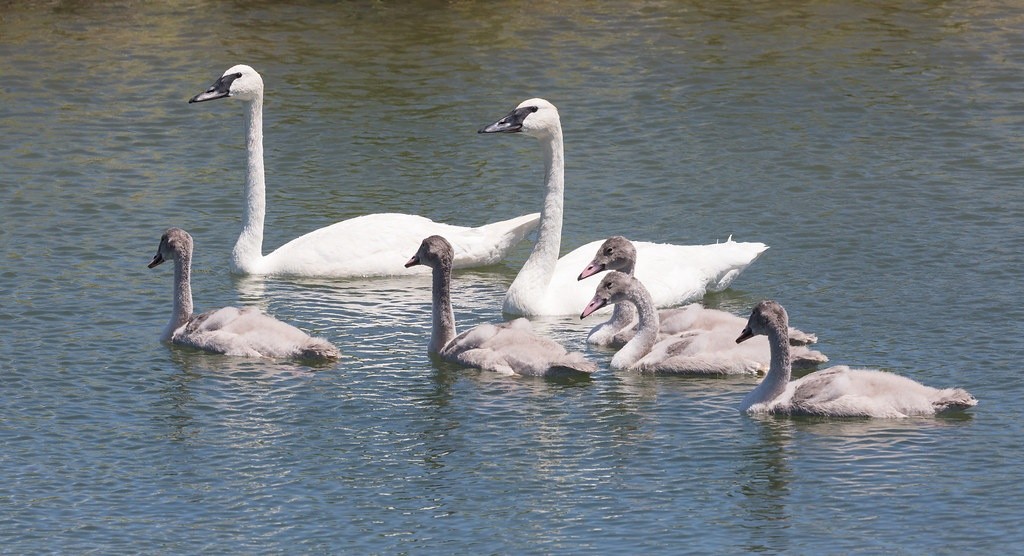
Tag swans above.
[147,228,340,362]
[404,97,979,421]
[189,64,542,277]
